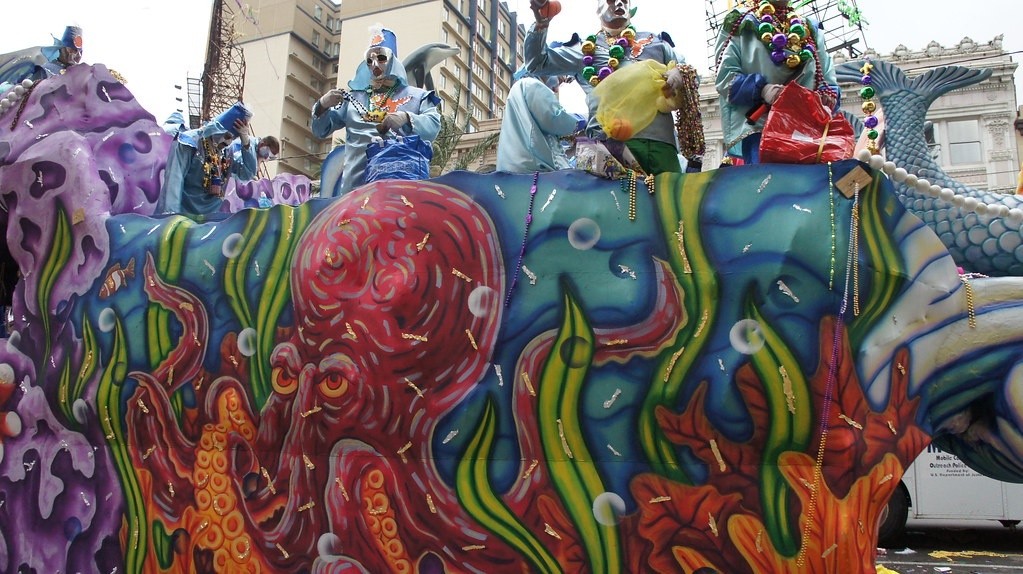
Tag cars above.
[874,442,1023,548]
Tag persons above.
[312,28,441,190]
[524,0,699,178]
[234,135,279,185]
[162,112,187,141]
[157,103,256,214]
[715,0,842,165]
[495,65,588,173]
[0,25,82,79]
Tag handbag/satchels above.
[575,132,648,180]
[364,136,433,184]
[593,58,676,141]
[759,80,855,165]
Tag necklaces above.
[963,277,976,328]
[621,168,655,220]
[9,77,42,130]
[504,171,538,308]
[861,62,879,155]
[675,63,706,158]
[333,88,374,123]
[716,1,838,98]
[581,24,637,86]
[795,161,859,566]
[368,80,401,111]
[204,137,231,198]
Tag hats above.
[62,26,83,50]
[370,29,397,58]
[168,112,185,122]
[215,103,253,137]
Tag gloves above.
[823,94,837,110]
[320,89,343,109]
[530,0,549,25]
[382,111,408,129]
[761,83,785,105]
[662,68,684,90]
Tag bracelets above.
[536,21,549,27]
